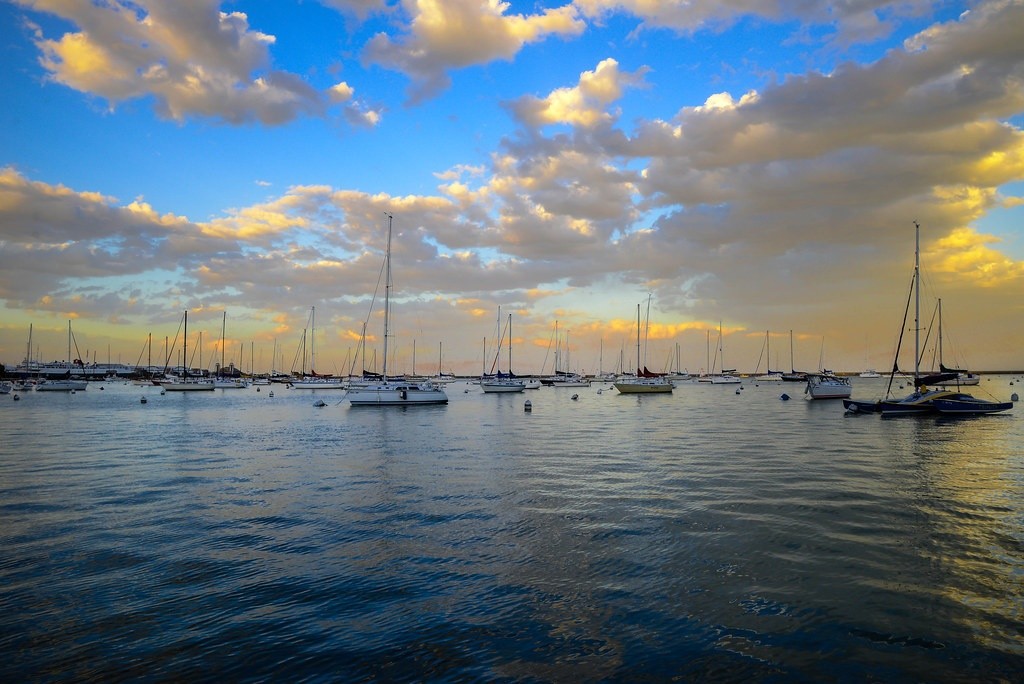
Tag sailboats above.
[0,210,1024,429]
